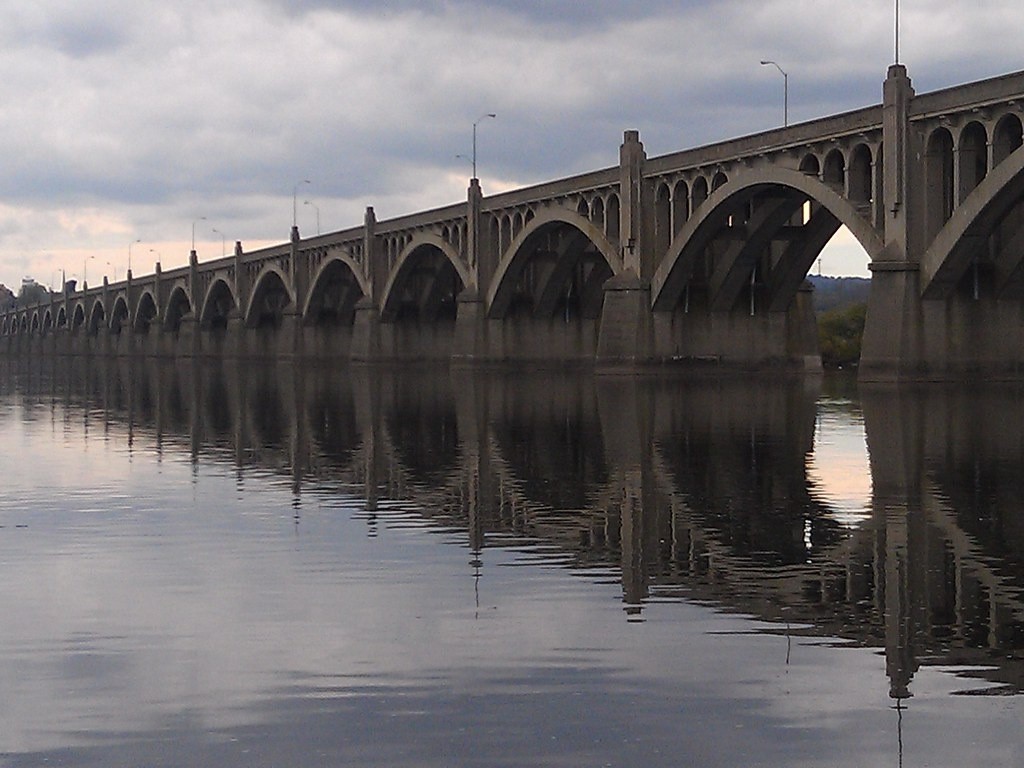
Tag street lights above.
[192,217,207,251]
[59,269,65,284]
[212,229,225,257]
[473,114,497,180]
[84,256,94,281]
[293,179,311,226]
[760,59,787,128]
[107,262,117,284]
[304,201,320,236]
[151,249,161,265]
[456,154,476,180]
[129,240,141,270]
